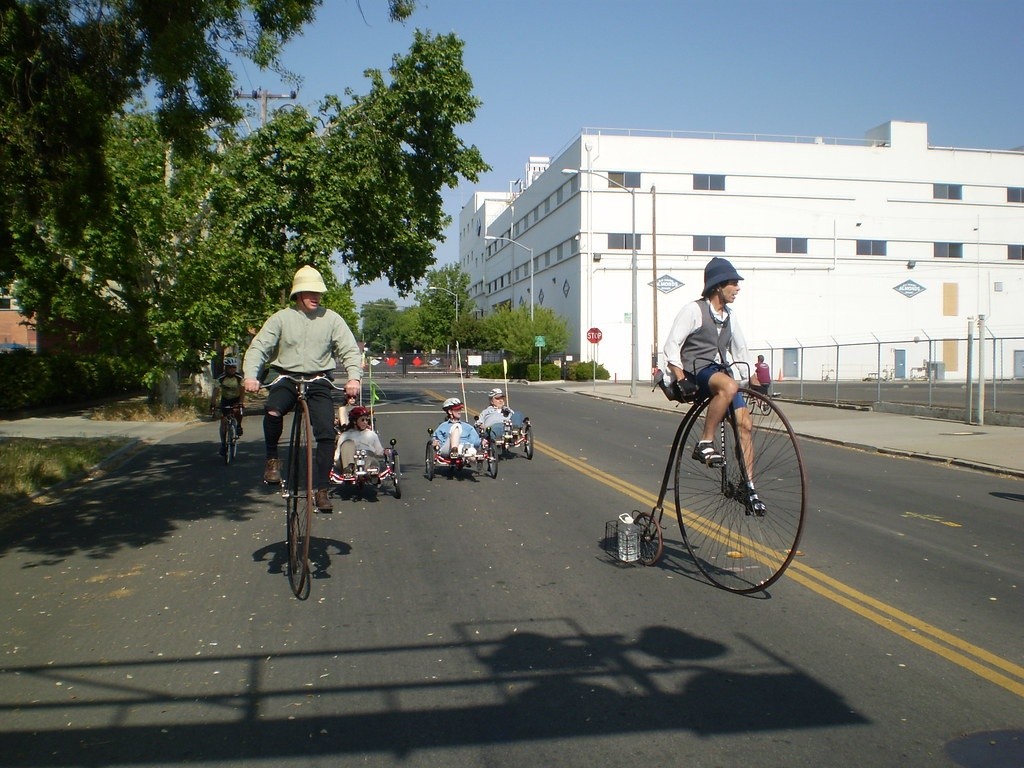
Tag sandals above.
[739,485,766,515]
[691,441,726,468]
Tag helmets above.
[349,407,370,420]
[224,357,238,366]
[488,389,503,398]
[288,264,329,302]
[442,398,461,409]
[701,257,744,297]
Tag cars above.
[428,357,443,365]
[862,372,886,382]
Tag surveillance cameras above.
[575,236,580,241]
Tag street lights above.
[428,286,458,371]
[484,236,533,321]
[914,329,931,407]
[561,168,637,399]
[979,315,986,425]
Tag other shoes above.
[369,460,378,484]
[220,443,226,456]
[343,472,354,480]
[237,426,243,437]
[450,451,459,459]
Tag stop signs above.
[587,328,602,343]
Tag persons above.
[209,357,250,456]
[748,354,771,409]
[664,256,768,517]
[241,265,365,511]
[431,398,489,467]
[333,391,391,483]
[474,388,525,446]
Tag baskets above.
[606,518,642,563]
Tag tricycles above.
[425,416,534,482]
[329,411,401,500]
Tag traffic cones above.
[778,370,782,382]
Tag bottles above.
[618,512,641,562]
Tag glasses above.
[358,419,369,423]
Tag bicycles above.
[260,374,346,596]
[604,357,810,594]
[210,404,246,465]
[746,390,772,416]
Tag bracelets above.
[348,379,360,383]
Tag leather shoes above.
[316,490,333,510]
[264,458,280,482]
